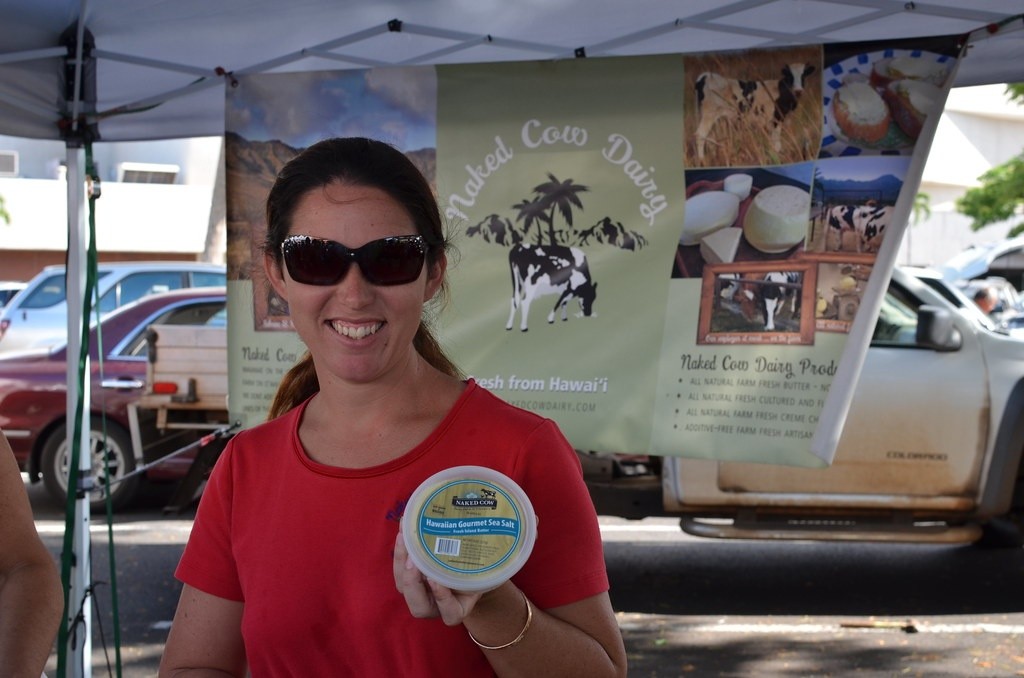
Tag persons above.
[0,430,64,678]
[156,134,629,678]
[973,286,998,314]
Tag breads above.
[834,56,942,142]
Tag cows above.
[822,204,894,254]
[716,272,800,331]
[692,62,817,168]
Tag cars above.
[1,282,228,517]
[546,236,1024,549]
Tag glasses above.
[281,235,428,286]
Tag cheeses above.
[679,184,811,265]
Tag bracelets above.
[468,589,532,650]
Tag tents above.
[0,0,1024,678]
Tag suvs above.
[0,260,228,366]
